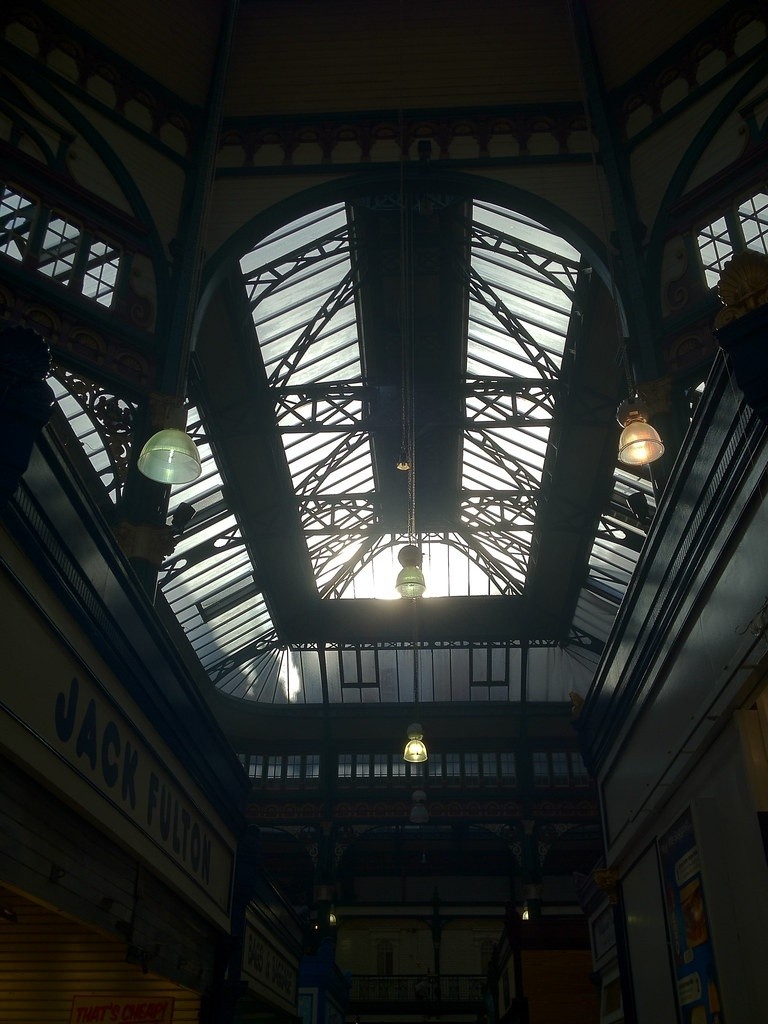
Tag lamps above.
[522,901,529,920]
[330,905,337,926]
[135,0,667,823]
